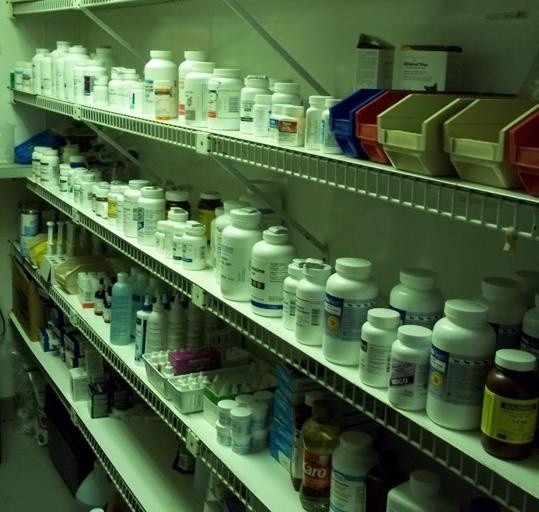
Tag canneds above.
[16,202,39,258]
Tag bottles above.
[521,291,538,357]
[144,277,167,299]
[214,198,244,276]
[268,82,302,138]
[13,60,24,90]
[22,62,33,91]
[135,185,165,245]
[229,407,252,434]
[109,271,131,345]
[57,164,68,192]
[93,277,112,323]
[68,155,87,168]
[236,393,254,407]
[303,96,331,149]
[386,324,431,413]
[319,98,345,154]
[386,469,459,511]
[209,207,222,243]
[252,93,271,136]
[290,391,321,491]
[182,220,208,270]
[7,69,14,88]
[196,190,224,245]
[82,60,105,106]
[321,257,379,367]
[95,188,109,219]
[238,75,269,135]
[249,228,294,318]
[512,268,537,291]
[479,347,539,462]
[143,49,176,117]
[154,79,173,120]
[79,174,95,208]
[93,78,109,107]
[281,262,309,332]
[31,146,38,177]
[154,220,167,249]
[183,60,216,127]
[388,265,444,330]
[247,400,267,429]
[206,68,242,132]
[134,294,151,362]
[278,105,305,148]
[151,349,174,379]
[214,419,231,447]
[51,39,71,99]
[108,65,122,111]
[250,424,268,449]
[238,180,281,228]
[62,146,77,164]
[186,299,203,351]
[129,266,140,284]
[63,47,90,99]
[474,275,525,349]
[358,308,402,388]
[73,60,86,103]
[121,68,138,110]
[128,82,142,114]
[41,149,60,187]
[425,298,497,432]
[93,45,118,80]
[177,50,206,122]
[35,146,51,182]
[171,220,186,260]
[164,189,192,220]
[254,389,275,406]
[133,273,149,326]
[107,183,123,223]
[122,178,151,238]
[164,208,189,255]
[66,169,81,199]
[30,47,50,92]
[298,399,341,512]
[293,260,332,347]
[40,52,48,92]
[329,430,378,512]
[116,195,123,227]
[217,399,234,425]
[90,186,98,213]
[145,295,168,355]
[229,429,251,456]
[220,207,261,303]
[168,293,186,353]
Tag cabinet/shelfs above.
[7,2,539,512]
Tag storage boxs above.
[509,112,539,198]
[10,253,46,340]
[357,90,408,164]
[377,95,529,176]
[444,97,539,188]
[328,89,388,158]
